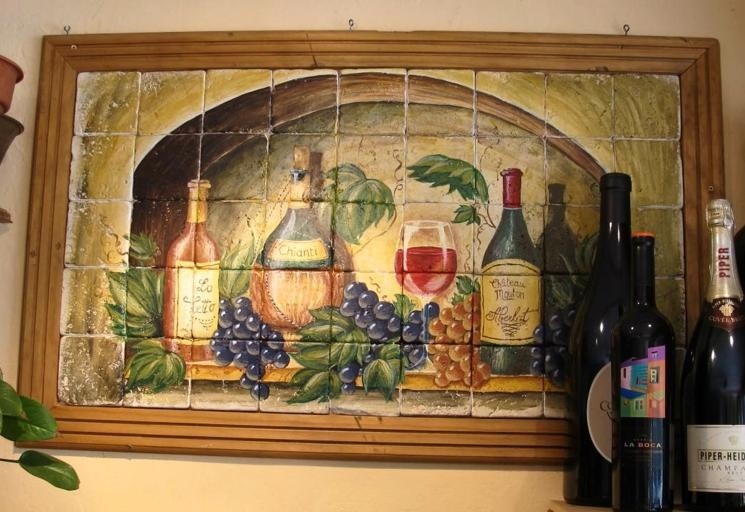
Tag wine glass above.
[393,218,458,376]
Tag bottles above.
[676,198,745,512]
[247,144,356,353]
[611,229,680,512]
[478,166,543,375]
[562,172,631,507]
[535,181,583,274]
[162,177,220,362]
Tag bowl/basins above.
[1,114,23,173]
[0,55,24,116]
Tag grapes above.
[209,296,290,401]
[339,282,437,397]
[530,310,575,390]
[428,292,493,388]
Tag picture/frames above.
[12,21,729,462]
